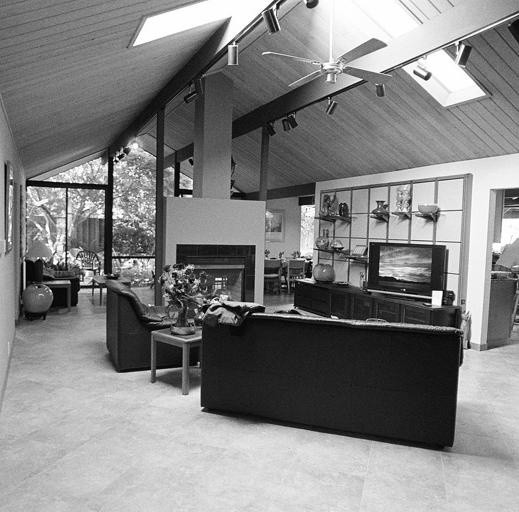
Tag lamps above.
[262,10,519,136]
[115,4,281,164]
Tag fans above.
[262,0,393,90]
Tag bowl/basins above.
[417,204,438,215]
[106,273,119,280]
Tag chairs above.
[25,259,80,307]
[287,260,305,295]
[75,250,101,296]
[106,279,198,373]
[264,260,281,296]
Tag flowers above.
[157,262,221,327]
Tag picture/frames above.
[264,208,286,242]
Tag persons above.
[495,238,519,277]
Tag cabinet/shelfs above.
[294,278,462,328]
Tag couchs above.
[201,312,464,450]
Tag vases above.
[373,200,387,215]
[170,325,195,336]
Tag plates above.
[336,281,348,286]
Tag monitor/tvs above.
[367,243,448,300]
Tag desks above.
[35,281,72,312]
[487,278,519,350]
[150,326,203,395]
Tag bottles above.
[320,192,347,218]
[316,228,344,251]
[372,200,387,214]
[360,271,368,291]
